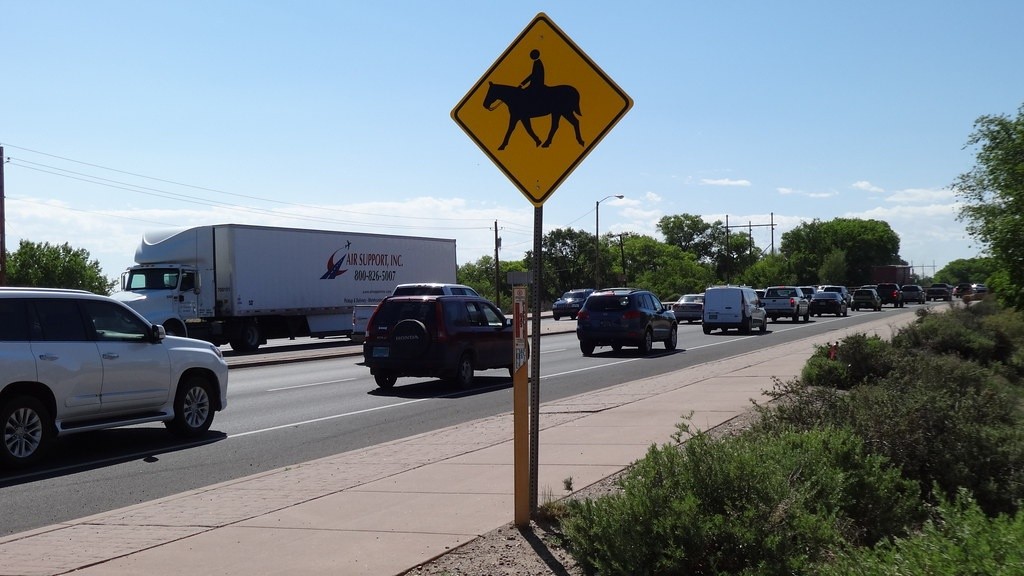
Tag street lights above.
[595,194,624,292]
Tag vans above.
[702,285,768,336]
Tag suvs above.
[392,283,503,326]
[901,284,926,304]
[797,285,852,308]
[0,287,230,472]
[850,289,882,311]
[877,282,905,308]
[576,288,677,355]
[364,295,531,391]
[860,286,878,291]
[551,289,596,322]
[953,283,988,299]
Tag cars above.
[673,294,705,325]
[808,291,848,317]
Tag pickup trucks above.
[759,285,812,324]
[925,283,952,301]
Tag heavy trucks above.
[93,224,457,354]
